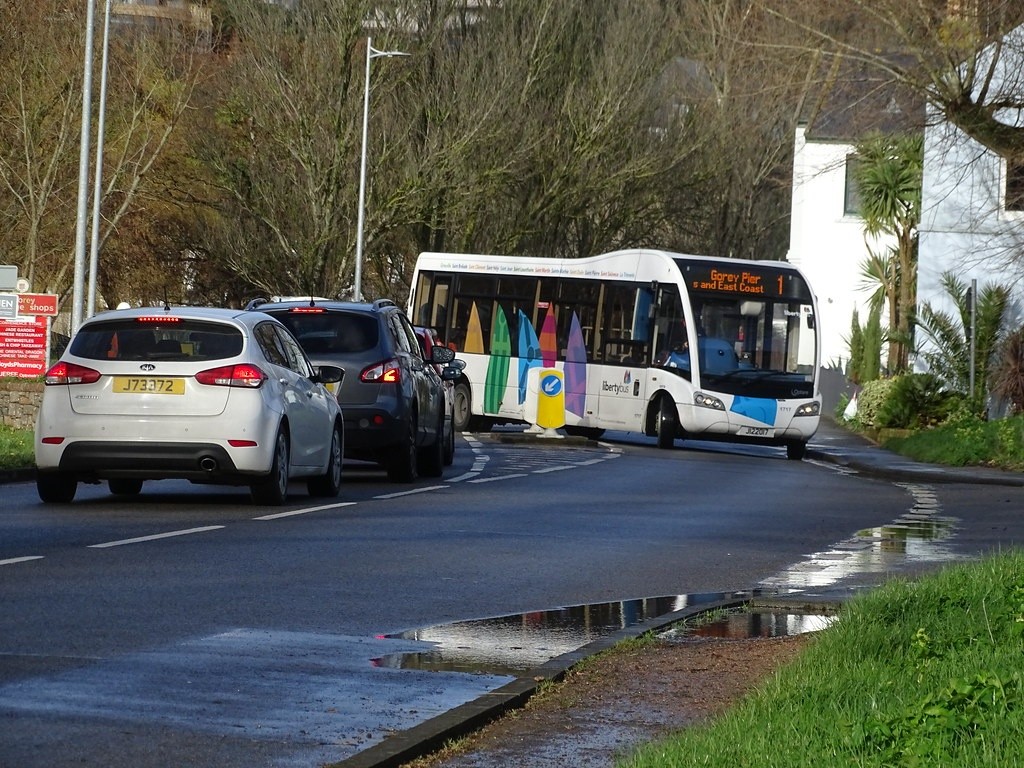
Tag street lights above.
[353,36,414,301]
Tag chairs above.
[126,329,184,358]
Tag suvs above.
[239,297,456,483]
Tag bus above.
[404,247,825,462]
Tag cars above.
[32,307,348,512]
[409,327,466,465]
